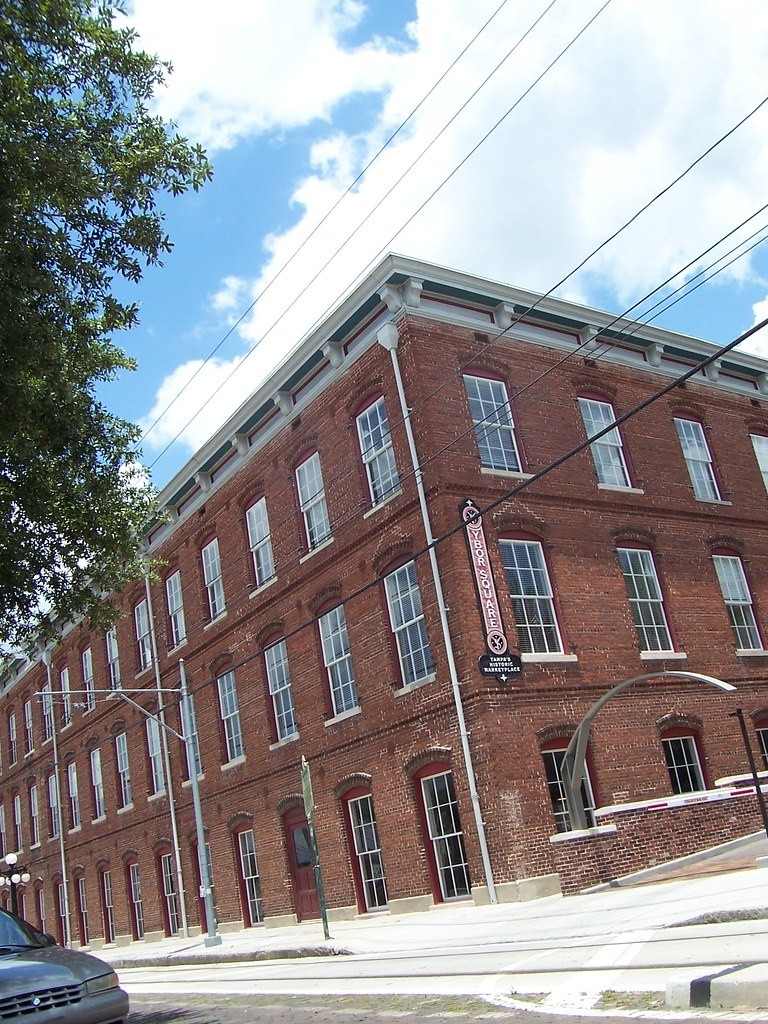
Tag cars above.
[0,906,130,1024]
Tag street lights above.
[1,854,31,917]
